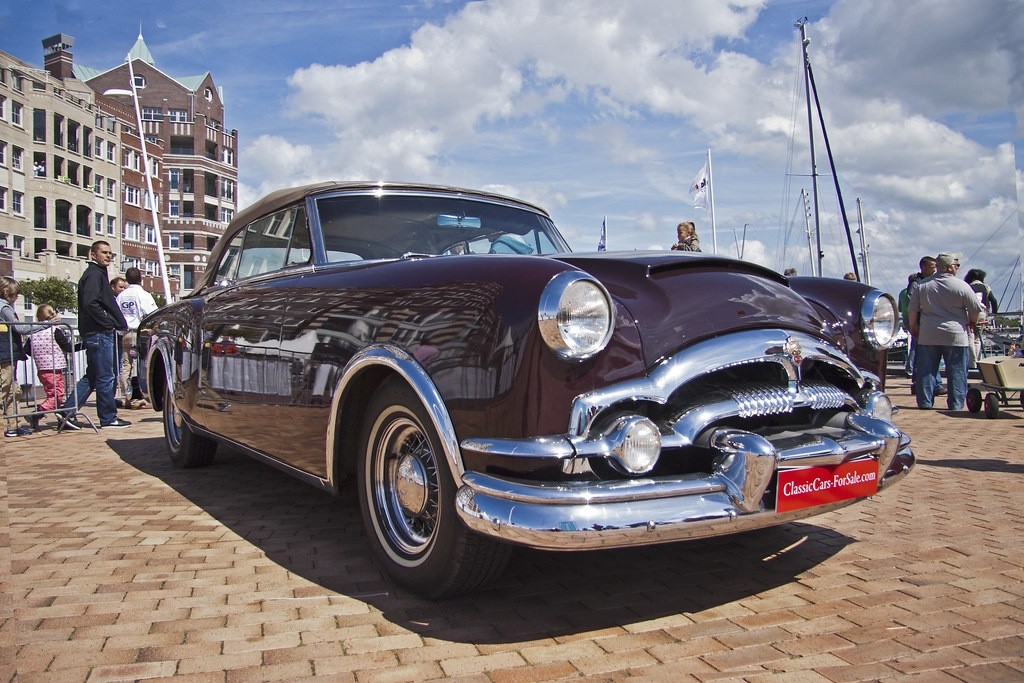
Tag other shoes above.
[142,398,153,409]
[221,404,230,411]
[935,385,948,395]
[312,394,322,404]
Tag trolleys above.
[966,319,1024,418]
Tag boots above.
[24,409,44,428]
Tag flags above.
[688,161,709,210]
[597,221,606,251]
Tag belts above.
[129,329,137,332]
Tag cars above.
[136,179,914,607]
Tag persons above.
[844,273,857,281]
[113,266,160,409]
[124,279,129,289]
[57,241,130,430]
[109,277,125,408]
[670,221,702,252]
[898,253,998,413]
[142,318,234,412]
[23,304,75,430]
[783,268,797,277]
[0,276,62,438]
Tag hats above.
[935,252,963,268]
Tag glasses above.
[951,263,960,267]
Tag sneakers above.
[56,412,81,429]
[58,413,82,430]
[101,418,132,429]
[5,427,31,437]
